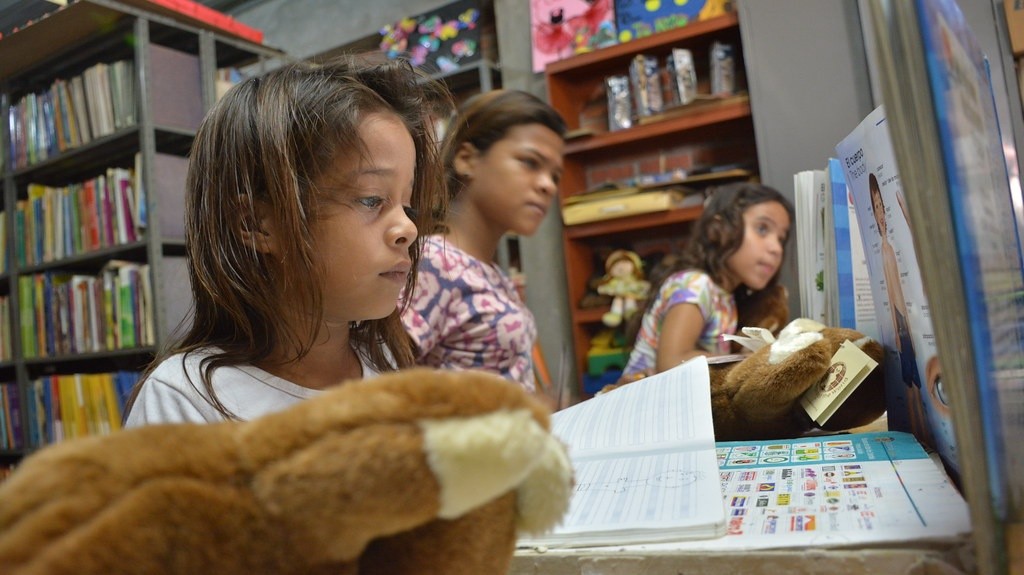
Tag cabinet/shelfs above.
[544,10,776,392]
[0,0,287,481]
[411,56,517,279]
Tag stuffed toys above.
[579,242,884,441]
[0,365,575,575]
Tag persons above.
[395,86,565,388]
[618,180,795,378]
[867,173,954,447]
[122,57,461,427]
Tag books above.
[513,356,721,549]
[794,156,874,344]
[0,62,158,486]
